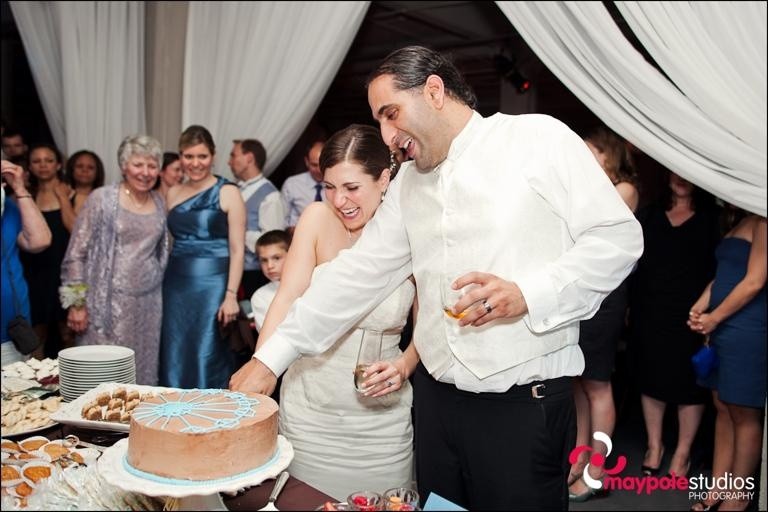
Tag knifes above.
[260,470,292,511]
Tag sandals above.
[689,491,755,512]
[642,444,664,475]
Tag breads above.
[82,388,154,422]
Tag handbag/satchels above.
[690,342,715,382]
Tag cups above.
[354,326,384,394]
[441,271,478,320]
[317,487,424,512]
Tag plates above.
[57,342,137,401]
[2,398,59,437]
[48,381,185,433]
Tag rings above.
[483,301,492,313]
[385,378,393,386]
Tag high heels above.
[569,472,612,501]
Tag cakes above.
[123,389,281,485]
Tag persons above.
[228,46,644,511]
[254,125,422,504]
[0,125,329,401]
[565,127,768,512]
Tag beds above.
[0,401,349,511]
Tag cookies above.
[2,357,61,435]
[0,436,84,508]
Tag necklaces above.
[347,227,358,243]
